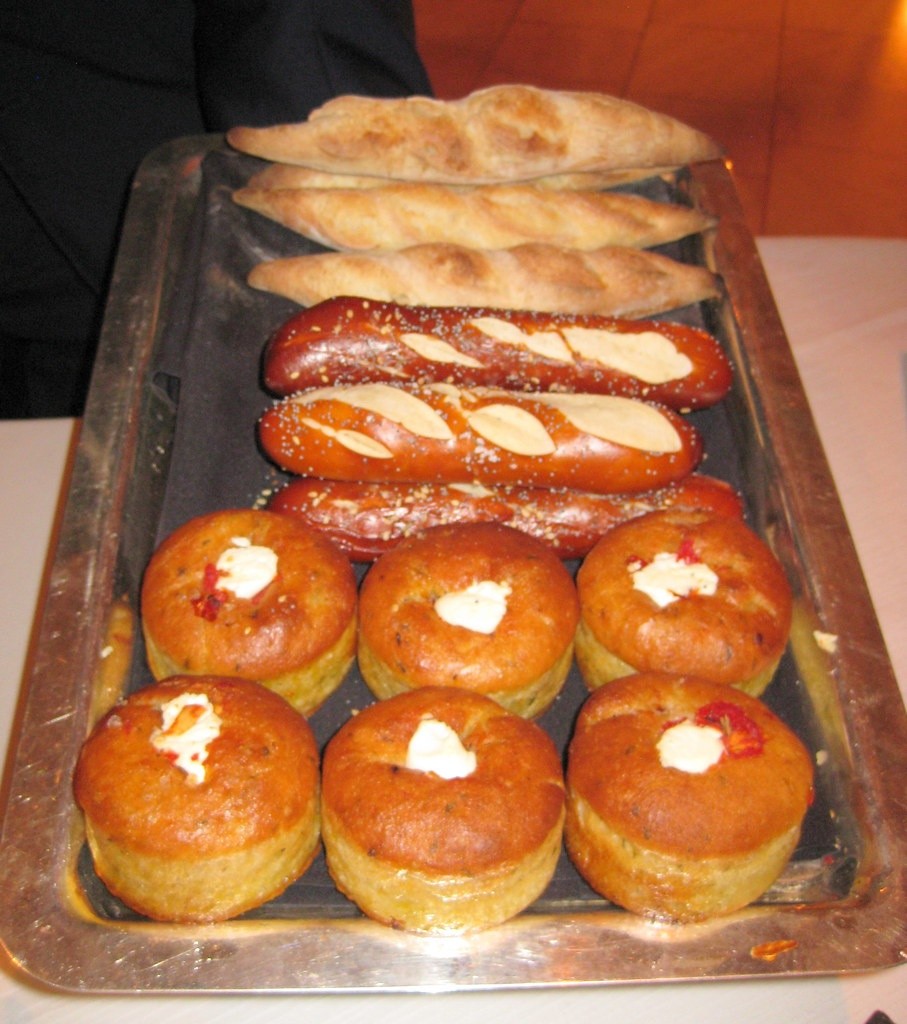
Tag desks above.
[0,234,907,1024]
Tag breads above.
[321,685,565,937]
[74,675,321,922]
[563,673,817,922]
[246,244,729,320]
[233,182,719,251]
[258,379,703,493]
[357,523,578,723]
[262,293,732,410]
[140,508,357,717]
[227,83,723,182]
[269,473,744,560]
[248,161,684,190]
[575,508,794,701]
